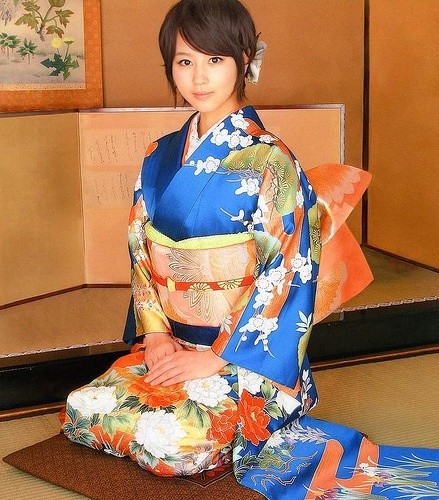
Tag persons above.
[67,0,317,479]
